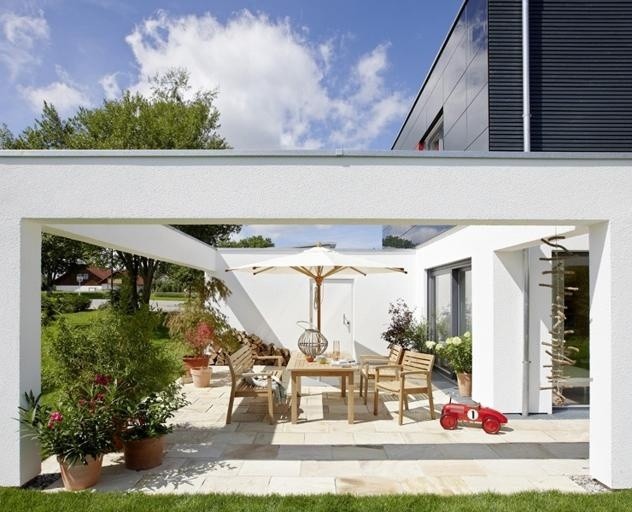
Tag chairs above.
[359,344,436,425]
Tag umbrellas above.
[224,241,409,355]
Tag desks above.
[286,350,359,424]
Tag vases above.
[456,372,471,397]
[57,453,104,490]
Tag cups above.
[332,340,341,361]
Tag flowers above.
[11,373,149,472]
[425,330,472,375]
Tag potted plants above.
[114,375,192,470]
[162,277,233,387]
[381,296,417,365]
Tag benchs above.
[226,342,284,425]
